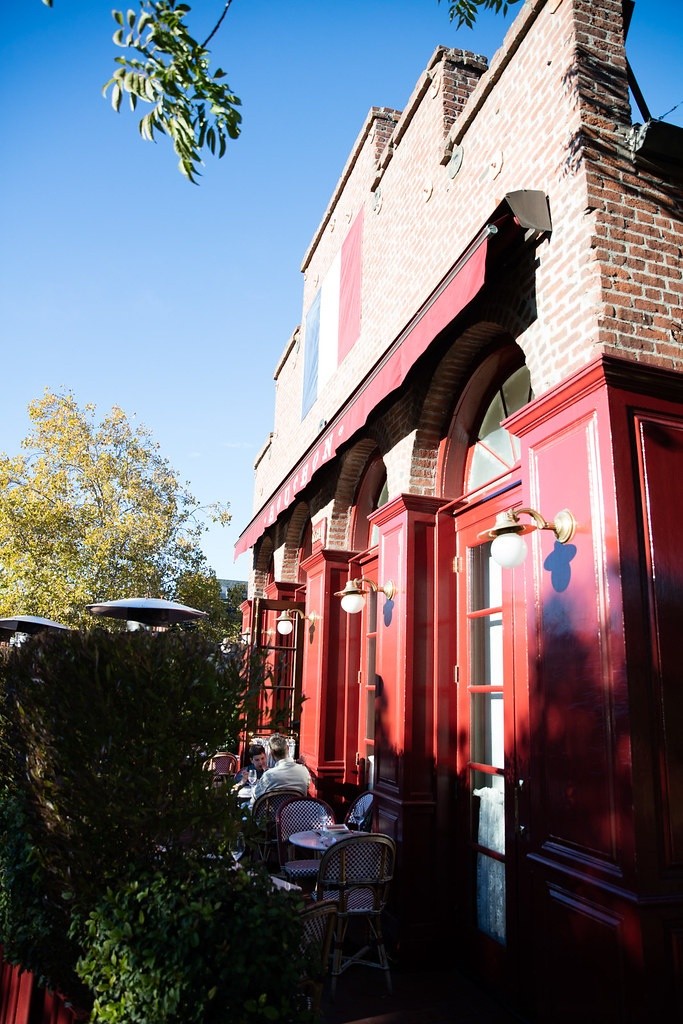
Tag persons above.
[250,734,310,803]
[233,744,272,791]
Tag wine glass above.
[248,770,257,789]
[318,805,328,840]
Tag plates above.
[321,824,349,833]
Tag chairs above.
[293,899,339,1021]
[312,834,397,997]
[276,797,336,888]
[252,788,306,868]
[202,752,238,785]
[344,790,377,833]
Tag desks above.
[289,827,371,943]
[228,786,252,803]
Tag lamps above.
[476,507,576,568]
[220,628,250,653]
[334,578,394,614]
[272,609,316,635]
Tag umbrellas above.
[0,614,78,636]
[87,596,207,625]
